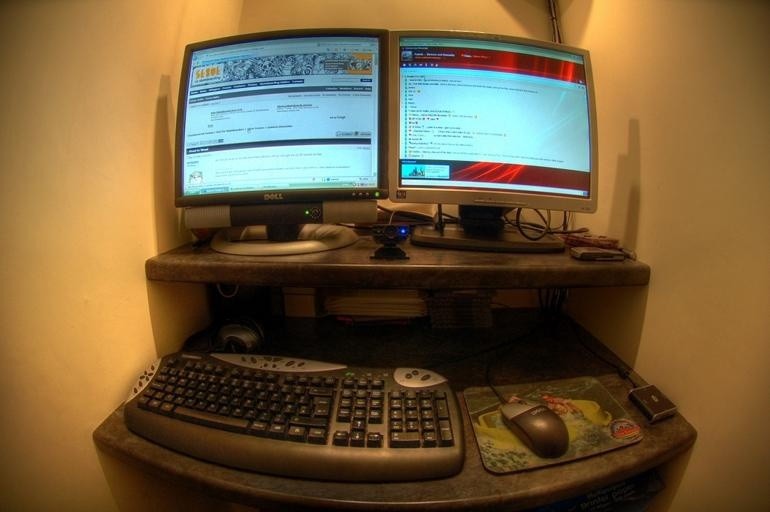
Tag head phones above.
[182,317,266,354]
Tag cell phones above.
[570,246,626,261]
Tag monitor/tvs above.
[389,29,598,253]
[174,28,389,256]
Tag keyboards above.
[125,352,464,482]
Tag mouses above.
[500,396,568,458]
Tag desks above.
[94,308,696,509]
